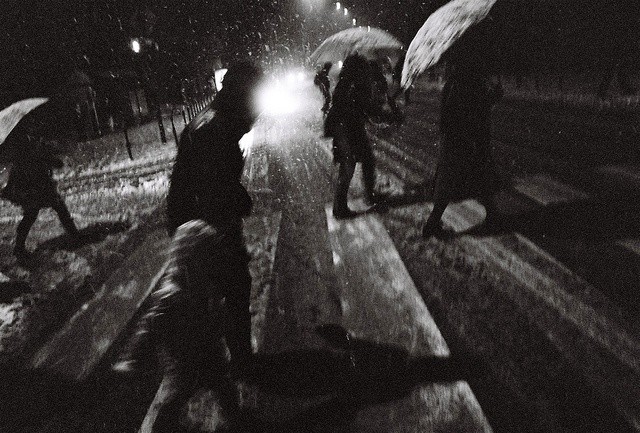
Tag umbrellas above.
[0,96,49,144]
[308,26,402,62]
[400,0,499,89]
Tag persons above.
[314,61,332,112]
[0,114,81,266]
[422,28,503,239]
[323,52,385,218]
[167,58,264,361]
[136,219,238,432]
